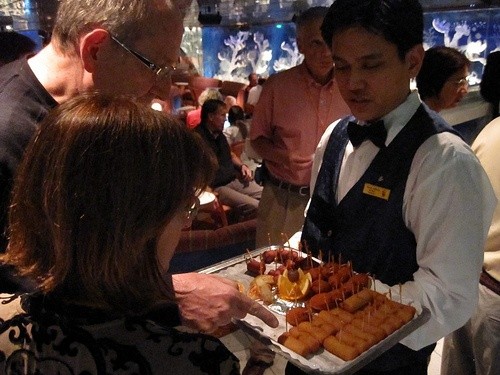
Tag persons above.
[0,96,264,375]
[415,46,472,115]
[0,0,279,338]
[228,106,258,181]
[285,0,499,375]
[249,6,347,248]
[193,98,263,215]
[442,114,500,375]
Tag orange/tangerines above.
[278,267,312,301]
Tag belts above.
[264,171,310,195]
[478,270,500,295]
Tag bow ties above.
[346,119,388,149]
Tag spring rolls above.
[246,230,416,361]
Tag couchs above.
[168,77,269,273]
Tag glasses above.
[109,35,175,77]
[444,78,467,86]
[177,197,201,226]
